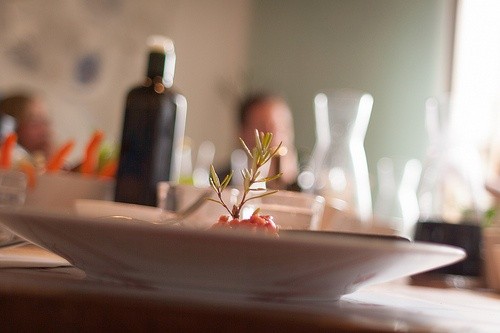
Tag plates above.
[0,209,468,304]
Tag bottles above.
[112,44,187,210]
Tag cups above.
[300,89,374,225]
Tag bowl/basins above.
[0,169,114,245]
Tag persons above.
[214,94,301,192]
[0,96,65,169]
